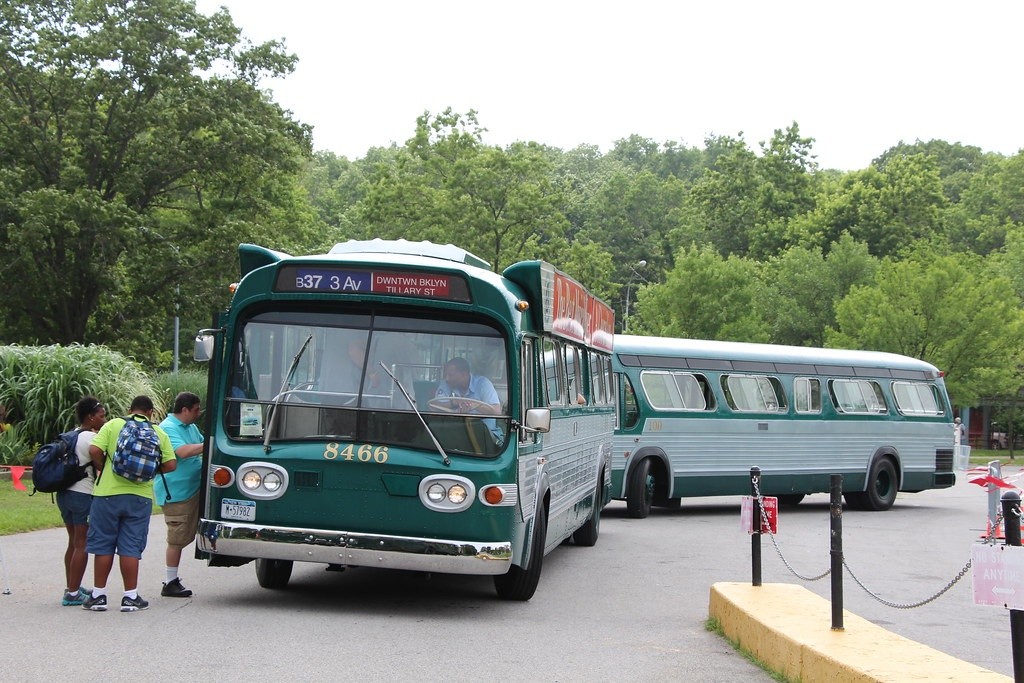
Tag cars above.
[990,422,1015,450]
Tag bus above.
[522,334,956,519]
[194,238,616,601]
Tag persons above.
[56,397,108,606]
[79,396,177,613]
[955,417,965,446]
[153,389,204,598]
[322,323,425,407]
[435,357,503,415]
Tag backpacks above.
[32,428,96,493]
[111,416,162,484]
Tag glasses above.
[92,403,100,411]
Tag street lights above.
[140,227,180,373]
[625,260,646,317]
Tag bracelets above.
[450,400,459,409]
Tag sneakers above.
[82,594,107,610]
[63,587,92,595]
[62,591,89,605]
[120,595,149,612]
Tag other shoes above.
[161,578,192,597]
